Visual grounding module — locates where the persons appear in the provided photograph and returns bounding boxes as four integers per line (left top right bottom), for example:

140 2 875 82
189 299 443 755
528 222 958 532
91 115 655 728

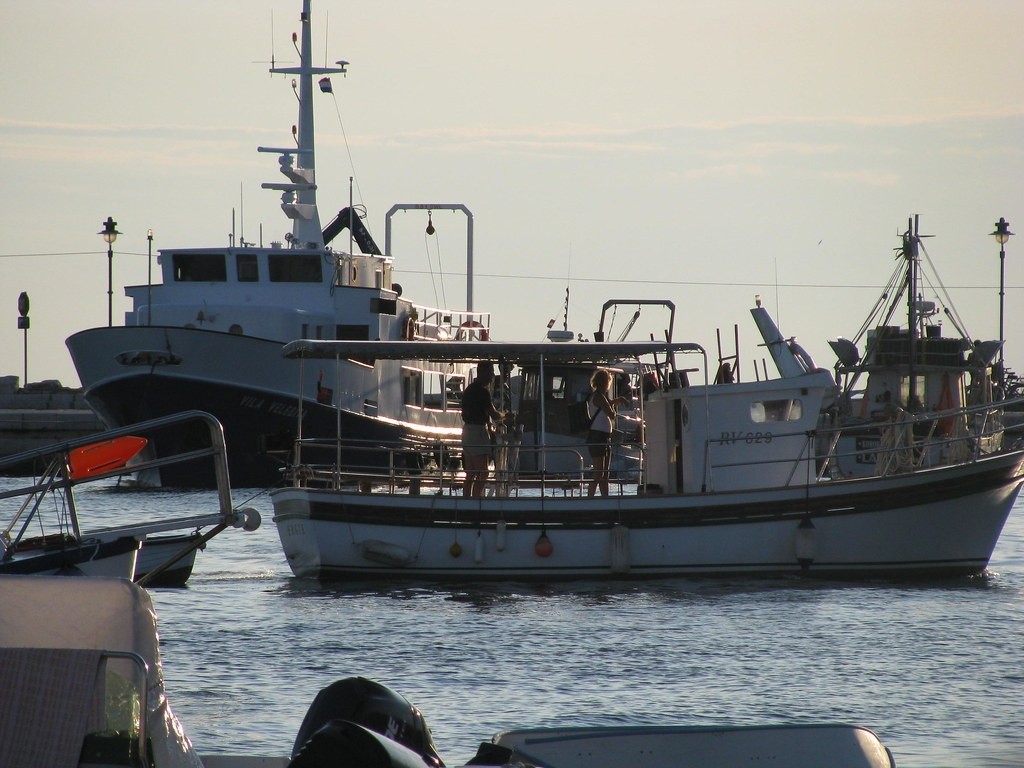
880 391 905 422
717 363 735 383
587 369 630 497
460 361 507 498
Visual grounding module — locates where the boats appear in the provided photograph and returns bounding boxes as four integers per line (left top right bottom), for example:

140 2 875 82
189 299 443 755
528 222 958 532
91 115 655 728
0 411 895 768
65 0 521 487
269 215 1024 581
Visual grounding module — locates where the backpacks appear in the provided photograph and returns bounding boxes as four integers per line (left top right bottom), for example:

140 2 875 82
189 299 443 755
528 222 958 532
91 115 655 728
567 393 601 433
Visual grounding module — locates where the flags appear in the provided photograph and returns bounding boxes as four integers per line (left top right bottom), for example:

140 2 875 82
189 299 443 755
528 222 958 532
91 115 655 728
319 76 334 93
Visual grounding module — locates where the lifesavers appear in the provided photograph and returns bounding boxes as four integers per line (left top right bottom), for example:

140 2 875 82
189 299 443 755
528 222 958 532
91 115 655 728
456 321 489 340
403 317 416 340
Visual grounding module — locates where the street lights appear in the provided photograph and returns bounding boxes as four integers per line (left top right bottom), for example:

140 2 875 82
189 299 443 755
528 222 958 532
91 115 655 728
987 218 1015 364
94 217 124 325
